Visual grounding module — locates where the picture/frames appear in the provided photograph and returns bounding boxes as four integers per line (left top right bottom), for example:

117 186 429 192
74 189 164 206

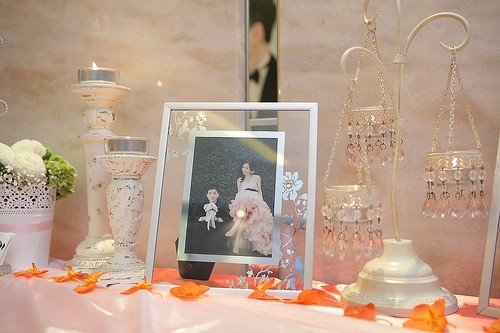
177 131 285 264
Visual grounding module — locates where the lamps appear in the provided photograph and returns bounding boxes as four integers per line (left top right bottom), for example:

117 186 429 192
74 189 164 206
321 0 487 319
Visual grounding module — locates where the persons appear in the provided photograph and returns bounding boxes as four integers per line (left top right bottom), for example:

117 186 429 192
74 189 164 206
190 186 232 255
224 160 263 255
248 0 278 130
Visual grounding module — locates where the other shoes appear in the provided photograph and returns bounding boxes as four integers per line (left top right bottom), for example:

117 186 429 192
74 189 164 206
233 242 239 255
225 229 237 237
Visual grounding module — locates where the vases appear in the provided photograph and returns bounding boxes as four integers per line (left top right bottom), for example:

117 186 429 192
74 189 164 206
0 184 57 272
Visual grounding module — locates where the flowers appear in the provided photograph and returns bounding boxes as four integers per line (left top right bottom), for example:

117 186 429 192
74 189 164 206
0 139 77 200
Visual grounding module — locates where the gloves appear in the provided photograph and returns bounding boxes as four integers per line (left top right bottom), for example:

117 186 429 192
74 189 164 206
203 203 218 212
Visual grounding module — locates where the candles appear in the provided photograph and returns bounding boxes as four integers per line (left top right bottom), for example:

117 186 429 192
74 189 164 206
77 61 118 84
107 137 148 154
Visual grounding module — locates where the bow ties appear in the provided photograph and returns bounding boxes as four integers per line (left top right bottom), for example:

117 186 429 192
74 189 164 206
250 70 259 82
